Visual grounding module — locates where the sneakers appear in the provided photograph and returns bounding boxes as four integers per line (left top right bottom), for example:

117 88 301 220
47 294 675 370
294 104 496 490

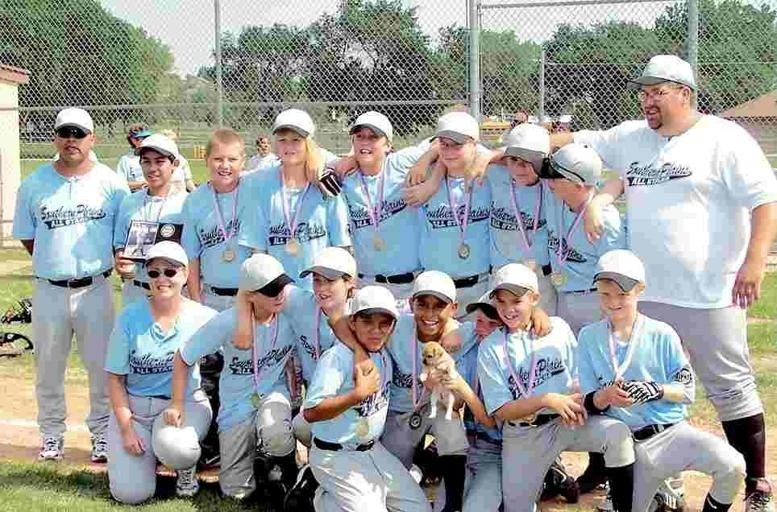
89 433 110 464
546 453 614 509
197 443 221 469
655 478 689 510
742 476 771 510
421 446 443 487
36 436 67 463
175 464 201 501
251 455 318 510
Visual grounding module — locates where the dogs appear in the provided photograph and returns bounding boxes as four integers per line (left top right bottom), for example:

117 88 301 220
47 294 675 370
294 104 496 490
419 341 456 421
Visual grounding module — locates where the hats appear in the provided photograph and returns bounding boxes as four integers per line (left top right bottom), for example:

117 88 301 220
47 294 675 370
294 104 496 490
299 246 356 281
591 248 647 293
348 284 401 322
143 240 189 270
239 253 295 298
626 54 699 96
466 263 539 319
410 270 458 308
128 128 178 163
54 108 94 136
428 112 481 144
272 108 315 140
500 122 601 187
348 111 394 143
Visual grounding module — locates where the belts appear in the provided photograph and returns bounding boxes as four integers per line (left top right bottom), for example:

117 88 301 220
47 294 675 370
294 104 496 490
134 280 150 290
313 436 384 452
488 264 552 278
357 273 414 284
453 273 479 288
48 268 114 288
631 423 671 444
509 412 560 428
211 288 237 296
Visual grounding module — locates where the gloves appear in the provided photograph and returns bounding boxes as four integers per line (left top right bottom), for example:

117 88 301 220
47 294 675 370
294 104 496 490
317 166 345 199
622 378 664 408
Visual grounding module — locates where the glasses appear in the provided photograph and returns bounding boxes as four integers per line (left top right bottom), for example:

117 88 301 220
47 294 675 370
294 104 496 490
145 266 183 277
58 127 87 139
636 86 684 102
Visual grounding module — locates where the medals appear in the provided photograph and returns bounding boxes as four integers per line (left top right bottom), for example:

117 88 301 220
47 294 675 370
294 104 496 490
605 312 642 409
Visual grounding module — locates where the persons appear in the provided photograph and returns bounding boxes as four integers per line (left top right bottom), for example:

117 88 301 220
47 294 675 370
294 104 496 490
553 52 776 512
9 108 635 512
575 250 748 512
